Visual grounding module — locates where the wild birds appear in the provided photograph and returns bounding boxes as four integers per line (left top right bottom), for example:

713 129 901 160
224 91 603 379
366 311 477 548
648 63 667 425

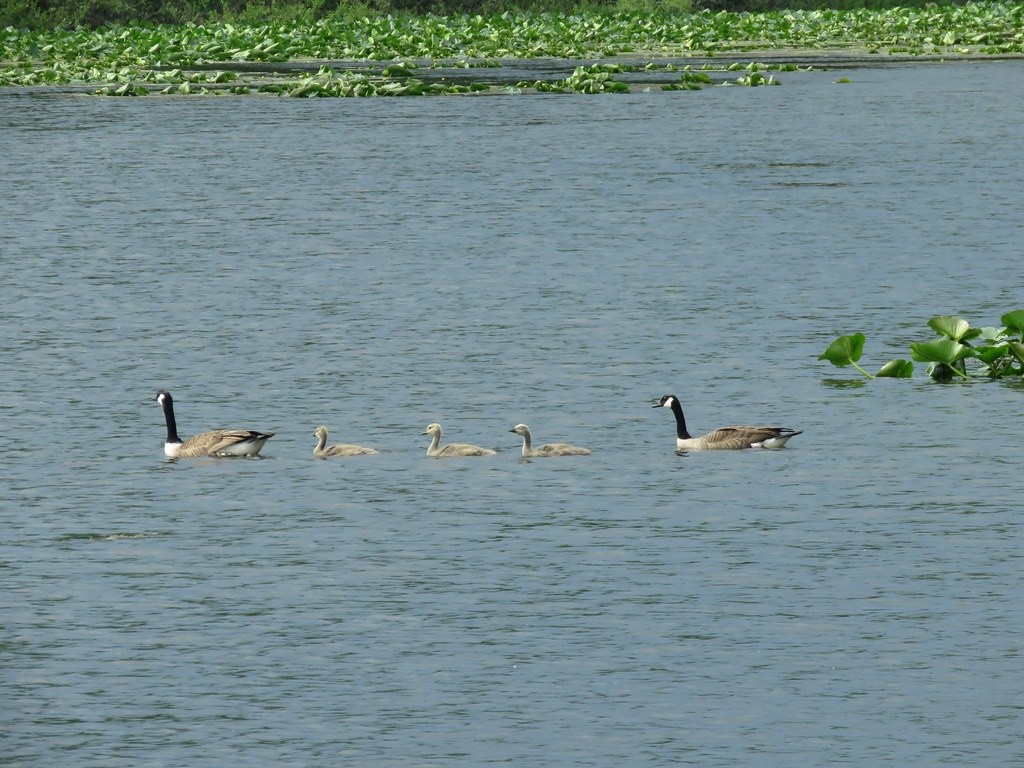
652 393 803 450
152 390 275 456
312 426 379 456
508 423 591 457
421 423 496 456
